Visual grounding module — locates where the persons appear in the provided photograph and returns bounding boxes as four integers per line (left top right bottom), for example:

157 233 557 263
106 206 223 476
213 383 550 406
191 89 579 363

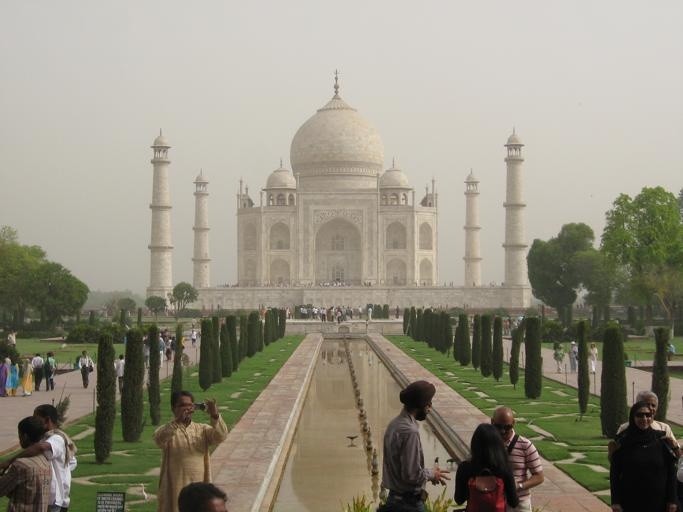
153 387 228 512
454 423 519 512
667 343 675 360
0 317 201 398
488 407 545 512
554 338 600 375
1 415 53 512
468 311 521 336
177 480 228 512
32 404 78 512
606 390 683 511
286 304 437 323
375 379 451 512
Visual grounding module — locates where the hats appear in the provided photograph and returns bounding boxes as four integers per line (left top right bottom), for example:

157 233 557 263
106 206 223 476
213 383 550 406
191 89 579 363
400 380 436 408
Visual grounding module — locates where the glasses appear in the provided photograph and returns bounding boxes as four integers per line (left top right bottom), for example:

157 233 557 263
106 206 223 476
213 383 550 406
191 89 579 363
493 423 514 430
634 411 653 418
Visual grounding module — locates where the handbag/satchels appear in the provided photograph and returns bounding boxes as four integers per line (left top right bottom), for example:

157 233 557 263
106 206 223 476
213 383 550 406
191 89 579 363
88 367 93 373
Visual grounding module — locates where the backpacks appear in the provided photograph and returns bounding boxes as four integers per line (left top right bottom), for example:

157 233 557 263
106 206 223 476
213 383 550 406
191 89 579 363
465 468 508 512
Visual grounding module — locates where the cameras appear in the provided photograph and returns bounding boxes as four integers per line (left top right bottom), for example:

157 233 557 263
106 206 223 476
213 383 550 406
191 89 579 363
191 403 205 410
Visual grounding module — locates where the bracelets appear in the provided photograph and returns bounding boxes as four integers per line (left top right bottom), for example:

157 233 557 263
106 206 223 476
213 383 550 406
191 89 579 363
519 482 524 490
